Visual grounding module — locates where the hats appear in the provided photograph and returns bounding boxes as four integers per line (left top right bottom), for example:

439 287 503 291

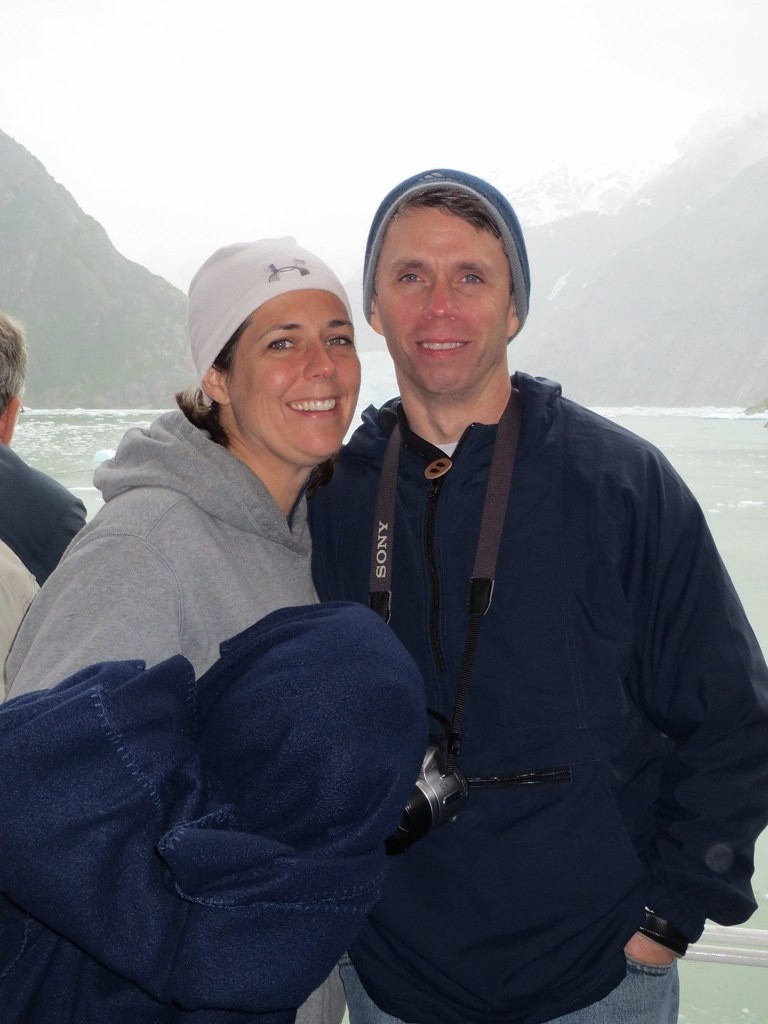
362 167 530 346
188 234 354 404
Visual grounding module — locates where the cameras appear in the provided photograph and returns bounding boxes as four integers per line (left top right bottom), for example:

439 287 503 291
392 746 469 848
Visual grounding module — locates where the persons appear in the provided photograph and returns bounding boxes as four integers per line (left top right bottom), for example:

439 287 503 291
304 169 768 1024
0 320 88 695
1 237 430 1024
